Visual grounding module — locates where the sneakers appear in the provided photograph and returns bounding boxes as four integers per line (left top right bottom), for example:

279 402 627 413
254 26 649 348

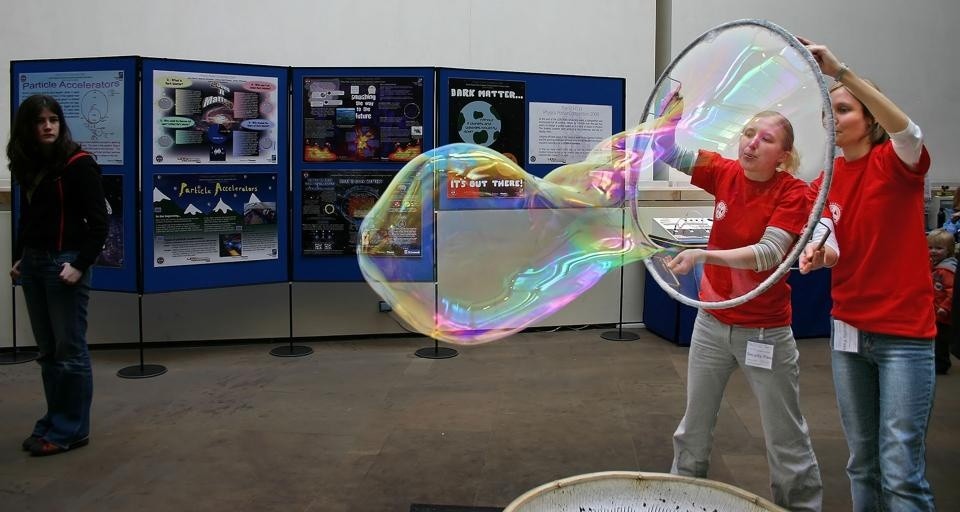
21 432 88 456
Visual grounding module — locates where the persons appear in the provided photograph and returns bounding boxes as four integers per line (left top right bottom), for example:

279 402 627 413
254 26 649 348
791 38 937 512
950 186 960 360
925 229 958 375
5 94 115 458
653 83 824 512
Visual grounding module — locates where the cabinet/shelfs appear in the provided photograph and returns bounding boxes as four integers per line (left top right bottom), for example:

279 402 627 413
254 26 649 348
643 249 832 349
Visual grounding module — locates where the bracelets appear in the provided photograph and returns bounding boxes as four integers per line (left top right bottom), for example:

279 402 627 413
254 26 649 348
834 62 847 84
